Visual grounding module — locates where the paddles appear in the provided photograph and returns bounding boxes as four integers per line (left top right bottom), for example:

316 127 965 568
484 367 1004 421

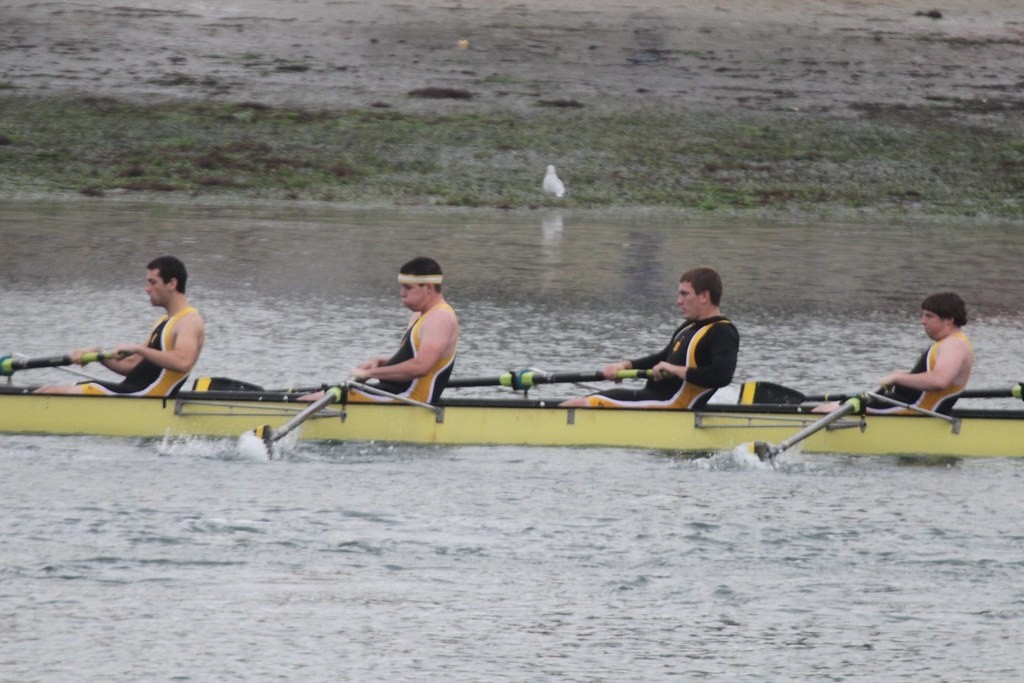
1 347 126 377
736 379 1023 404
253 375 367 462
747 384 893 464
444 365 671 390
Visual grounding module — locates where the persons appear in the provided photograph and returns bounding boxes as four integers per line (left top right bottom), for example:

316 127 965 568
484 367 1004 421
559 265 740 410
33 255 205 397
809 292 974 418
296 257 459 407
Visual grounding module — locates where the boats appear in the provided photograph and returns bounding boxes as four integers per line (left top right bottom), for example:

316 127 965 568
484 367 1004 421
0 383 1024 462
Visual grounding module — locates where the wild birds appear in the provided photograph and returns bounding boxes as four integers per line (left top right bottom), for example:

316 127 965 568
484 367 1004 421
542 165 565 200
